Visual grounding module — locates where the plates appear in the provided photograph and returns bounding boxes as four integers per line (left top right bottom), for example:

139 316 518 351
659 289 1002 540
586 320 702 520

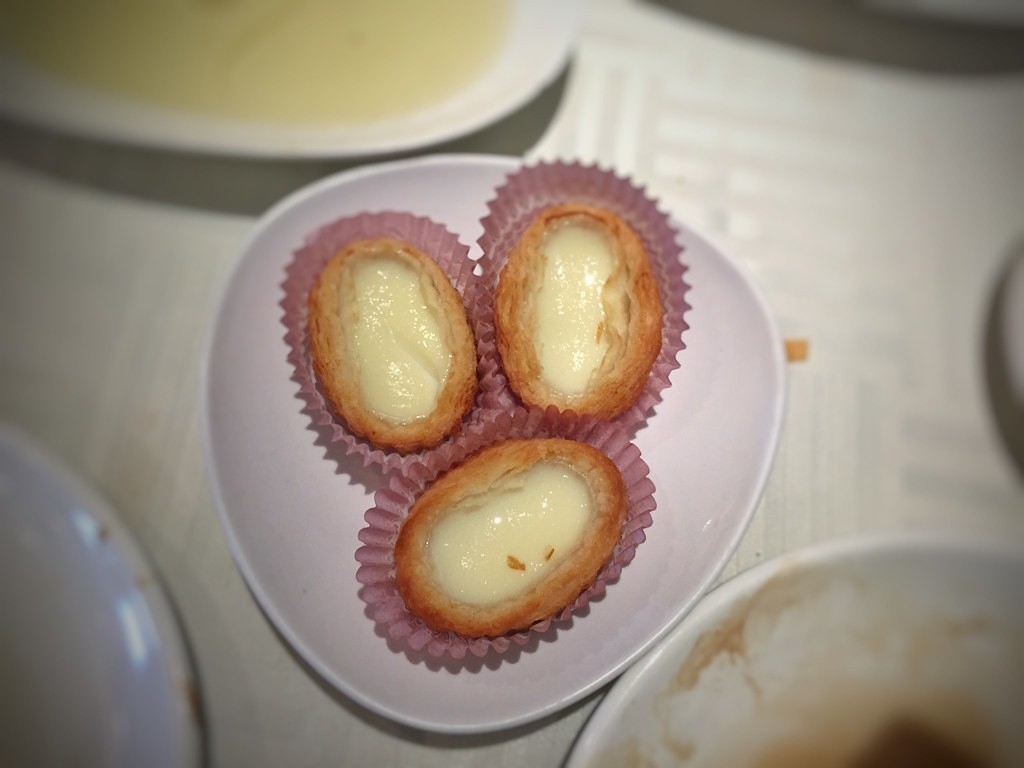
0 426 207 768
0 0 575 160
201 154 788 735
563 532 1024 768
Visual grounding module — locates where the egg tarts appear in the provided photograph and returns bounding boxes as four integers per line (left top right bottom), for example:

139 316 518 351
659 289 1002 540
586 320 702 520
305 236 475 451
394 439 627 638
493 209 660 421
